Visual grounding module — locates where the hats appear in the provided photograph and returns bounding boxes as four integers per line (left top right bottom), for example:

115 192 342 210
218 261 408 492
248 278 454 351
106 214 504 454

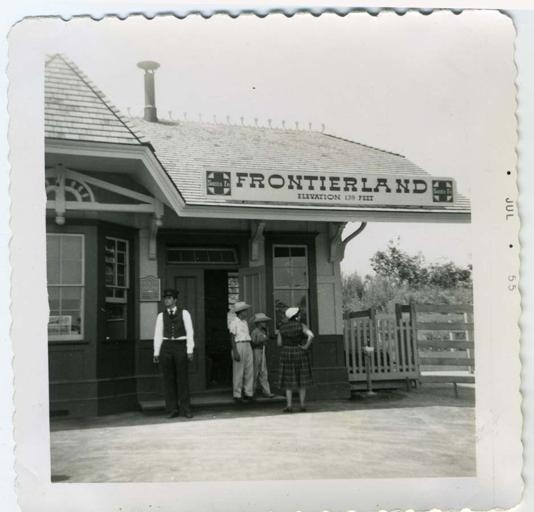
232 301 251 314
253 312 272 323
163 289 179 298
285 307 300 319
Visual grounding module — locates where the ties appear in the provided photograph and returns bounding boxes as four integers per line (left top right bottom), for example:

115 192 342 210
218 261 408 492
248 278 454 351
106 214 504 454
170 310 173 318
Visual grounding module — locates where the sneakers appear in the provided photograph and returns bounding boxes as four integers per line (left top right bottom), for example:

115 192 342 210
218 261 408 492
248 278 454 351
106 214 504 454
283 407 307 412
262 393 275 398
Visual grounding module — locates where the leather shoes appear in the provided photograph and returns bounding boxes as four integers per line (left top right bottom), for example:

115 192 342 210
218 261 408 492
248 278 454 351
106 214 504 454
183 408 193 418
167 409 179 418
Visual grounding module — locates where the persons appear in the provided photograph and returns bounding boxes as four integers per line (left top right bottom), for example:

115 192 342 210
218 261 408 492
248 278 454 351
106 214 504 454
228 300 274 404
272 305 317 413
152 288 197 420
